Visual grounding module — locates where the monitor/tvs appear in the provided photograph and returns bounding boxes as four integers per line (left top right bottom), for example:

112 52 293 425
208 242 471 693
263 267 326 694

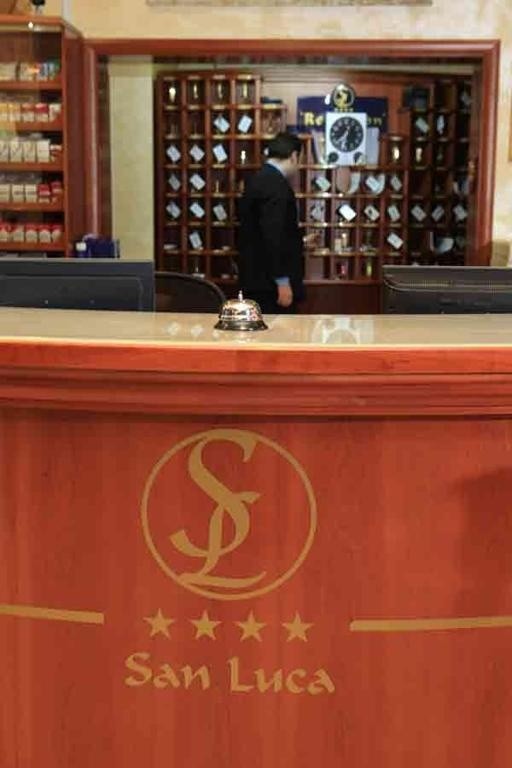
381 262 512 315
0 259 158 311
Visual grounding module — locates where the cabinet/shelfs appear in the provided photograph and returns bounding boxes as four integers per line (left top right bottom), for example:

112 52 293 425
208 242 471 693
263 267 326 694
0 13 87 259
156 71 479 281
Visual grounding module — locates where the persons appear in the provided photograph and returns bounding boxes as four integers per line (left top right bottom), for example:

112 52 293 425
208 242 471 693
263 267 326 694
239 132 305 314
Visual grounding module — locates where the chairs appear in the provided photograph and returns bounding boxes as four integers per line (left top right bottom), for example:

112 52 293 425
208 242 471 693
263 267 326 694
154 272 227 313
